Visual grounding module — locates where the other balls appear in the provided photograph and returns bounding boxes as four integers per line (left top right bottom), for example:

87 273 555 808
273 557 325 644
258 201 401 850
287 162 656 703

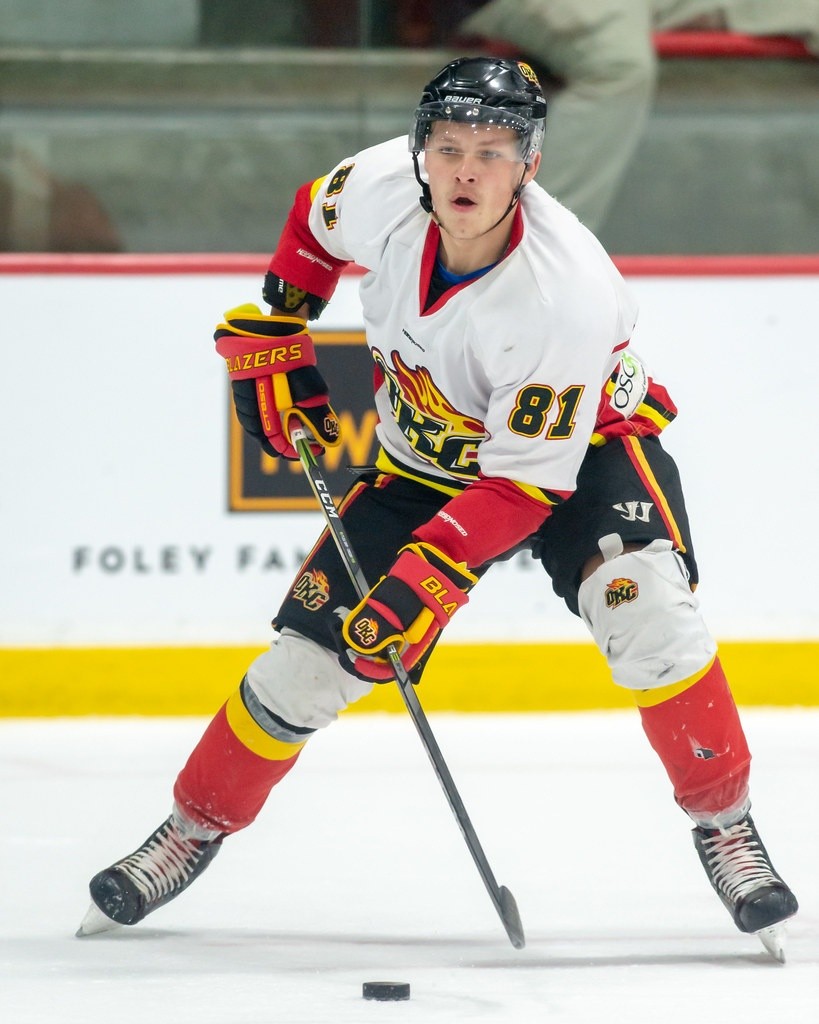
361 980 413 1002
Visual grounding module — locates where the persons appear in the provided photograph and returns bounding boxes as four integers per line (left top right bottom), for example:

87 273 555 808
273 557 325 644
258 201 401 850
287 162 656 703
423 1 659 235
78 53 802 967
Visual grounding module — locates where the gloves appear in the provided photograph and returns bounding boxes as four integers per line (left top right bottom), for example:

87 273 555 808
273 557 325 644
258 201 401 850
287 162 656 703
327 541 479 685
214 302 342 461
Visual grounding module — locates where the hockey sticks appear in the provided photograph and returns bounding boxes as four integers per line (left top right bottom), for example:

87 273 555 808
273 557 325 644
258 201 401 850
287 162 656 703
287 411 527 952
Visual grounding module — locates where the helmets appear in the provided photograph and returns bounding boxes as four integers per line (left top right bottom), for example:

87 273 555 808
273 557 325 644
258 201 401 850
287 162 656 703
411 55 548 168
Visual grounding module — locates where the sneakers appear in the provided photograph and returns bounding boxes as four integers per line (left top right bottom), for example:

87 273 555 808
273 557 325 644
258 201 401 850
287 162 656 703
75 814 222 937
691 813 799 964
